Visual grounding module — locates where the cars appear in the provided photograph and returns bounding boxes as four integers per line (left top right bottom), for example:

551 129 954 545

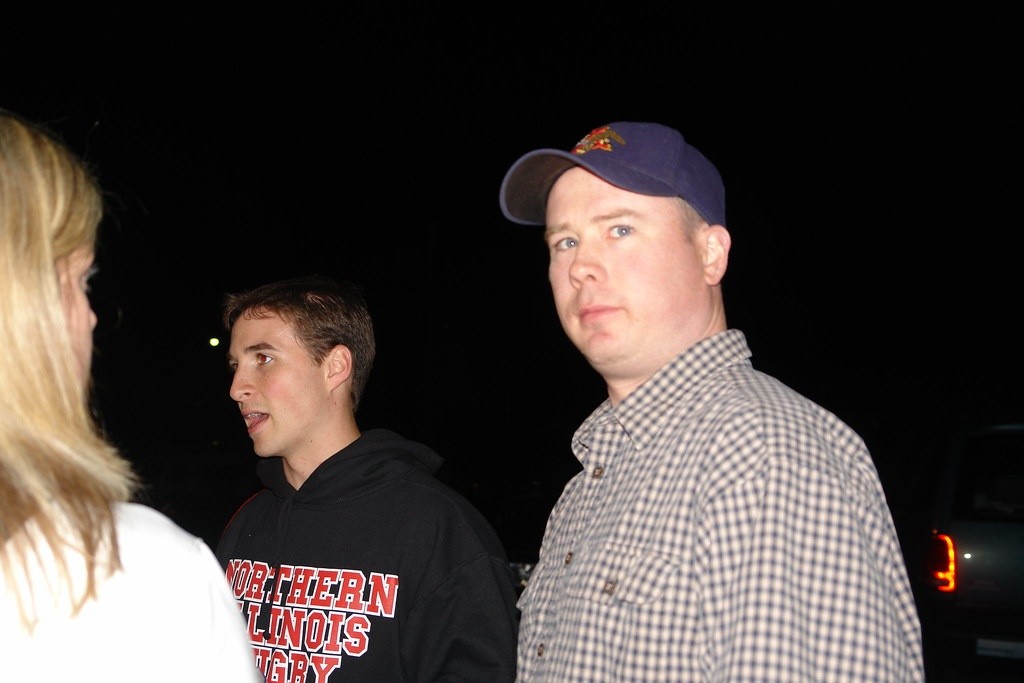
926 423 1024 666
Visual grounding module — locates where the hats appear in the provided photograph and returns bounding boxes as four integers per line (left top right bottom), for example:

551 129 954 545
501 124 726 225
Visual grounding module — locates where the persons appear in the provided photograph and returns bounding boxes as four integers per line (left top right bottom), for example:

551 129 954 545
499 122 925 683
0 115 269 683
216 274 520 683
